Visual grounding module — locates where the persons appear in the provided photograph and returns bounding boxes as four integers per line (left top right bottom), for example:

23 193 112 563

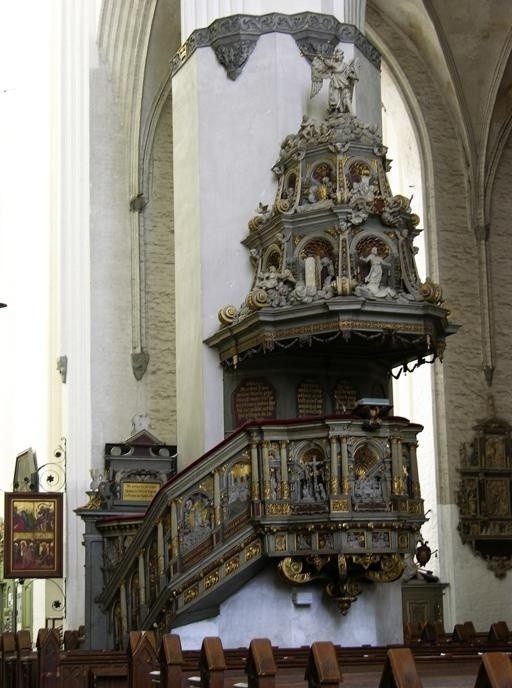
318 48 360 113
320 256 334 286
359 246 392 291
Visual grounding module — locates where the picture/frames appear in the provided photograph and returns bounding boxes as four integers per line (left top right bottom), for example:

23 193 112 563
4 492 62 578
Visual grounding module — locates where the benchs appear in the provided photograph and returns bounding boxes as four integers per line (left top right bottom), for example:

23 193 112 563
0 628 510 688
